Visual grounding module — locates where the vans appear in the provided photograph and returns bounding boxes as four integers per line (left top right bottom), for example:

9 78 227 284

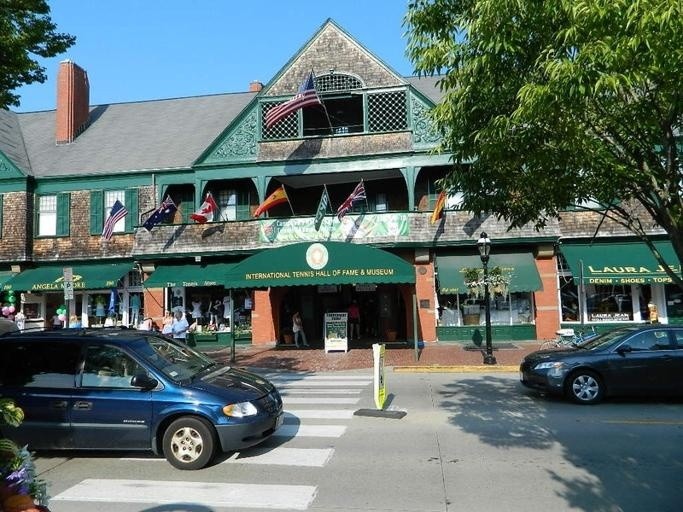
0 323 284 470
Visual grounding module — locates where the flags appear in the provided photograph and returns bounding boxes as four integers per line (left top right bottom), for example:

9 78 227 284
190 194 217 225
336 182 365 223
314 189 328 232
429 190 446 226
142 196 176 232
101 200 128 240
107 287 120 317
253 186 288 218
264 73 321 129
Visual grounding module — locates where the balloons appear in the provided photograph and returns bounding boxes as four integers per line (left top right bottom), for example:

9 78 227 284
1 289 15 316
56 303 68 320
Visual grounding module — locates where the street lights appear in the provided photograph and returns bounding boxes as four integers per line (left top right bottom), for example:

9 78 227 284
475 232 498 367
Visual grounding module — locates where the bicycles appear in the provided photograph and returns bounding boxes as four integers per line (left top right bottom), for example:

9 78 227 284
536 330 584 352
557 325 600 345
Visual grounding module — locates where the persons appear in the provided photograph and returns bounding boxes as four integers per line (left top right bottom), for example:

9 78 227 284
291 309 310 349
347 299 361 341
207 299 221 330
129 293 142 326
222 296 233 326
94 295 107 317
49 315 63 330
170 310 190 345
68 312 81 329
14 308 27 331
190 299 203 319
364 298 377 335
172 289 183 308
161 311 173 337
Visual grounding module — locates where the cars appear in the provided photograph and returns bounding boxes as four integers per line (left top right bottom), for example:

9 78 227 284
515 322 682 406
